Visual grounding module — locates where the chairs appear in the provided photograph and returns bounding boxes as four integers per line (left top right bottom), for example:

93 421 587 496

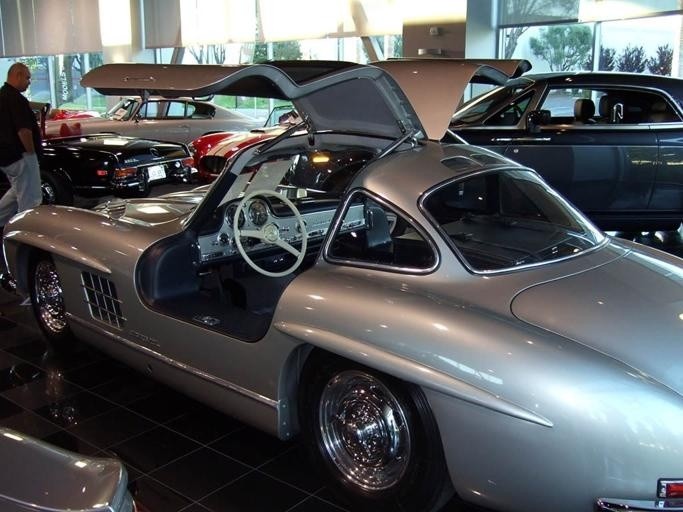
574 96 667 124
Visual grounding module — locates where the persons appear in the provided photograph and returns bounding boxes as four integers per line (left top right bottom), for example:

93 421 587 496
0 61 46 233
